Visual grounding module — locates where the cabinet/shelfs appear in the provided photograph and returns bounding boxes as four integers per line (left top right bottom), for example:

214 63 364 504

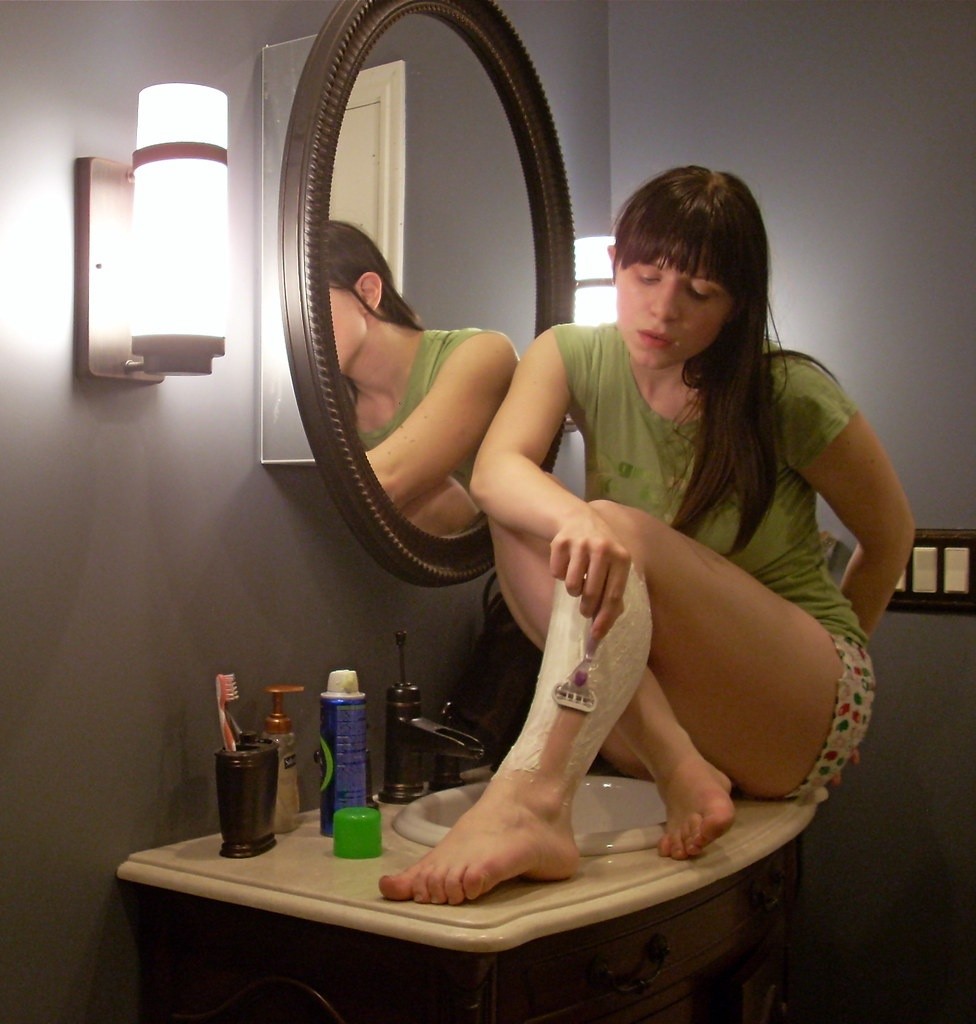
142 835 805 1024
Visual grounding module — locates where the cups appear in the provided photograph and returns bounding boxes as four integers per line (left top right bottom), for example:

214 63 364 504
214 739 280 858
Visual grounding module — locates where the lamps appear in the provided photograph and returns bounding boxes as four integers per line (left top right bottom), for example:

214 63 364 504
572 237 618 326
73 82 229 382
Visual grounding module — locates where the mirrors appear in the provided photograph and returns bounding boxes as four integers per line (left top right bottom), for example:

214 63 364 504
279 0 576 590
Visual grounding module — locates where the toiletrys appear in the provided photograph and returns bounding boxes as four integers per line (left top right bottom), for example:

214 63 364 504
262 680 306 834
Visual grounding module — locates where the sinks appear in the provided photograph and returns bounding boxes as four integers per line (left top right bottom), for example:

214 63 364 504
391 769 701 852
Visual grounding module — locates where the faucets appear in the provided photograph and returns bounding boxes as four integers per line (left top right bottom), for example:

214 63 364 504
378 628 484 806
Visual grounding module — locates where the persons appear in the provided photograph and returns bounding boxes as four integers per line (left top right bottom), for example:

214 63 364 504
377 163 917 904
328 221 517 540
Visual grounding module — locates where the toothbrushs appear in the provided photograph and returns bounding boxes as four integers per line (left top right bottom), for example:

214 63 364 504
214 671 240 753
